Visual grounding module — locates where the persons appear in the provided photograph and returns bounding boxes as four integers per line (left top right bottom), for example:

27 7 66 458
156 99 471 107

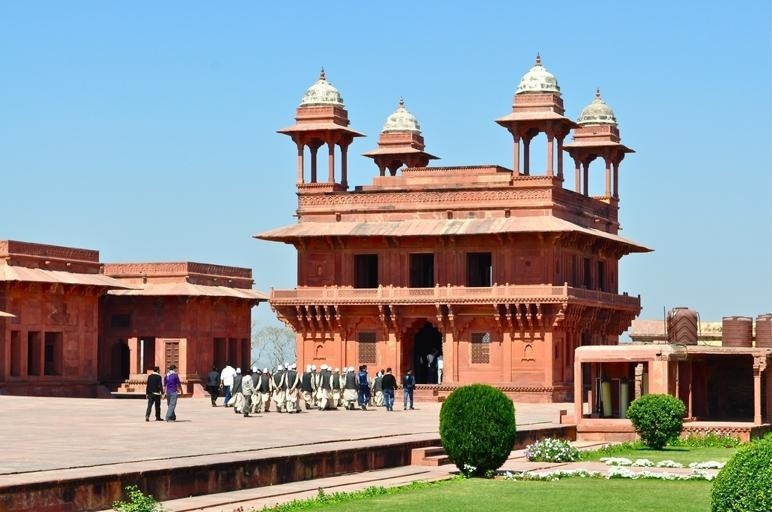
420 351 444 385
202 359 419 417
144 365 164 424
162 363 184 423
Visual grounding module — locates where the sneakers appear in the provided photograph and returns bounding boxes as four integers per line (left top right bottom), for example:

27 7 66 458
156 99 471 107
234 402 374 418
403 406 415 410
386 405 393 411
145 416 150 422
155 418 163 421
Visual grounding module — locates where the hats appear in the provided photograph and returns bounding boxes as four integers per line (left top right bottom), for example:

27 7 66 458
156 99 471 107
235 363 355 373
169 364 178 370
